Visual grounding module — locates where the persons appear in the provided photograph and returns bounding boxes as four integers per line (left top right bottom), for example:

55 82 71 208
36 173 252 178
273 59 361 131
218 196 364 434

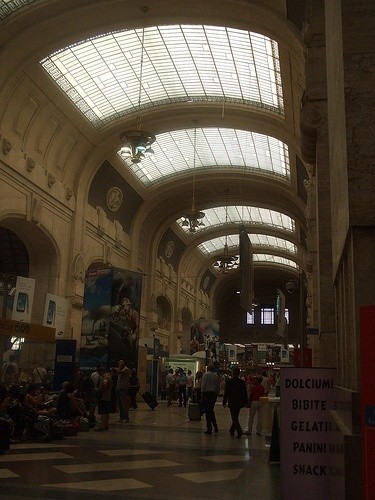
0 354 20 392
129 368 141 409
89 364 113 428
31 361 47 391
165 367 203 408
18 369 32 396
44 366 55 390
112 360 131 423
200 363 221 435
218 366 233 397
238 369 255 401
223 368 248 439
261 373 277 397
111 291 139 355
198 321 210 344
245 374 266 436
208 335 221 362
74 363 88 391
0 380 89 451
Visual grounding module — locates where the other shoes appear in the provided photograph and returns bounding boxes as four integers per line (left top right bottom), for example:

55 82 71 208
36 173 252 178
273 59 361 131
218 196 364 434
118 417 129 423
256 432 260 435
231 432 234 436
204 431 212 434
236 432 243 439
244 432 251 435
93 422 109 431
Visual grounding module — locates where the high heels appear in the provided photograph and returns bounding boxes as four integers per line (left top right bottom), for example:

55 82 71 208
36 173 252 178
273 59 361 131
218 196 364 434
12 433 27 441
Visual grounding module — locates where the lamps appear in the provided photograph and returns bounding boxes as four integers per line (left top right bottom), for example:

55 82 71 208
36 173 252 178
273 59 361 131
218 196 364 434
118 6 156 164
181 121 205 233
213 206 238 274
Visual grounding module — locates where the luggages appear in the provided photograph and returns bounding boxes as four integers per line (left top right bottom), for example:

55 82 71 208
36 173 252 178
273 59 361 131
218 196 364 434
138 392 158 411
188 391 202 421
